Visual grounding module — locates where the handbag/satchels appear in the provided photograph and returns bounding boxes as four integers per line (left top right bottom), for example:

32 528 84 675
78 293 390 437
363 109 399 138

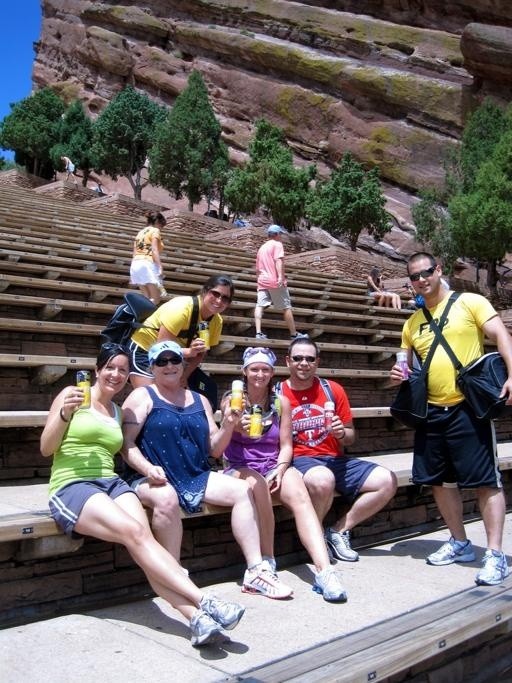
456 351 512 420
187 295 219 413
389 366 429 429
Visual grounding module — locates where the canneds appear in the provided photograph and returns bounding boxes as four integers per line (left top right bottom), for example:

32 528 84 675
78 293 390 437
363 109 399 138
396 351 410 381
324 401 336 431
199 320 210 351
76 370 92 409
231 380 244 412
248 404 262 440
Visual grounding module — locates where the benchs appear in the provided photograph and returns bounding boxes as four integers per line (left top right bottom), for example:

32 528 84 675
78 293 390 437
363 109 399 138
0 181 512 683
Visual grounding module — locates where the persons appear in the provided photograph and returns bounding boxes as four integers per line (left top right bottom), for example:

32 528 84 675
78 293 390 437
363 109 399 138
253 223 309 339
129 210 165 303
95 182 102 193
368 265 390 308
378 273 403 309
272 337 399 561
38 341 246 647
407 279 450 310
120 338 294 599
60 155 78 188
127 273 234 389
390 252 512 584
219 346 348 601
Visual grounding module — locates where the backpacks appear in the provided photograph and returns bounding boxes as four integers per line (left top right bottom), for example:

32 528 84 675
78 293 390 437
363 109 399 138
97 290 214 346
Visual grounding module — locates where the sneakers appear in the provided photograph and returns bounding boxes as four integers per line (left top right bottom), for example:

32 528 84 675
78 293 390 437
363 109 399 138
255 333 268 339
198 593 245 629
475 549 509 585
426 537 476 565
290 332 310 340
189 610 230 646
238 527 359 602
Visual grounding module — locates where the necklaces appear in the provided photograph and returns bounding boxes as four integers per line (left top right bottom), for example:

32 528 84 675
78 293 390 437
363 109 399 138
246 392 269 402
160 386 182 404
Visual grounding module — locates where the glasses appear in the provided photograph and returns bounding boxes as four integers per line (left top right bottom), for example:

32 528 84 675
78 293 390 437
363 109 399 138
245 347 274 359
291 356 316 362
210 290 232 305
151 355 182 367
409 266 437 282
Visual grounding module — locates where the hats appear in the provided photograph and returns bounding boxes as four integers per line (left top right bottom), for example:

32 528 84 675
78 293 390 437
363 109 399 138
241 347 277 368
267 225 286 235
149 341 184 366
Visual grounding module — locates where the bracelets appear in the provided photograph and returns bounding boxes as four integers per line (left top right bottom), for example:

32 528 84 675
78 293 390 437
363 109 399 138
336 431 345 439
280 466 285 475
60 408 71 423
